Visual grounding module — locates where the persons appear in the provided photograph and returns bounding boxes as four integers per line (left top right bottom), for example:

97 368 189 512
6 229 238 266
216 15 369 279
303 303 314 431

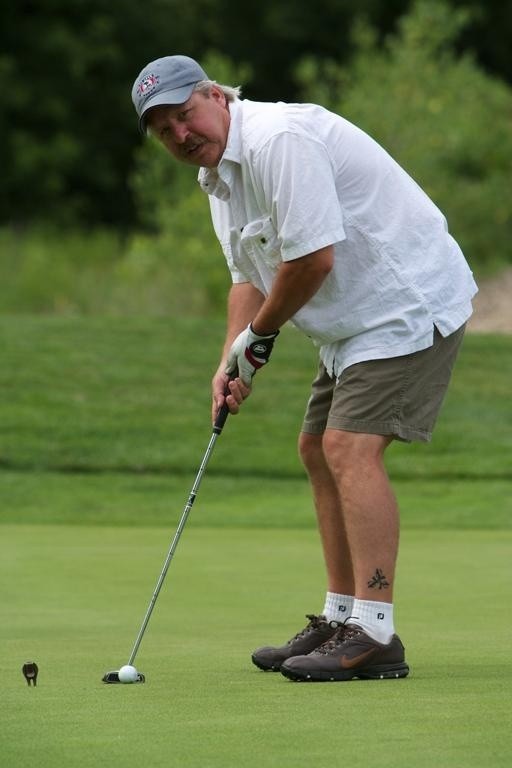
132 55 479 682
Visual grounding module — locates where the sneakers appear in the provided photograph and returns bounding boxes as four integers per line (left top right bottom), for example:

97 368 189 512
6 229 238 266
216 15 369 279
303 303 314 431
252 615 409 682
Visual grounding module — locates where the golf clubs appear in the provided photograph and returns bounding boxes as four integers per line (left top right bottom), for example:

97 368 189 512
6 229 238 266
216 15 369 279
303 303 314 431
102 367 239 684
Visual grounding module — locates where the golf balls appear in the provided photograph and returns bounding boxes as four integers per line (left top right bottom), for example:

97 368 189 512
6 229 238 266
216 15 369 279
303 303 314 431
118 665 137 683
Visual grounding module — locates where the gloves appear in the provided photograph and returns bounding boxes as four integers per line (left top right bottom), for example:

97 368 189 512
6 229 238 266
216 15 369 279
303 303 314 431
224 320 280 387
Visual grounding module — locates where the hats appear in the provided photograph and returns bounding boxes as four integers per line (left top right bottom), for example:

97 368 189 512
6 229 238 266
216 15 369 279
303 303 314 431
131 55 210 137
